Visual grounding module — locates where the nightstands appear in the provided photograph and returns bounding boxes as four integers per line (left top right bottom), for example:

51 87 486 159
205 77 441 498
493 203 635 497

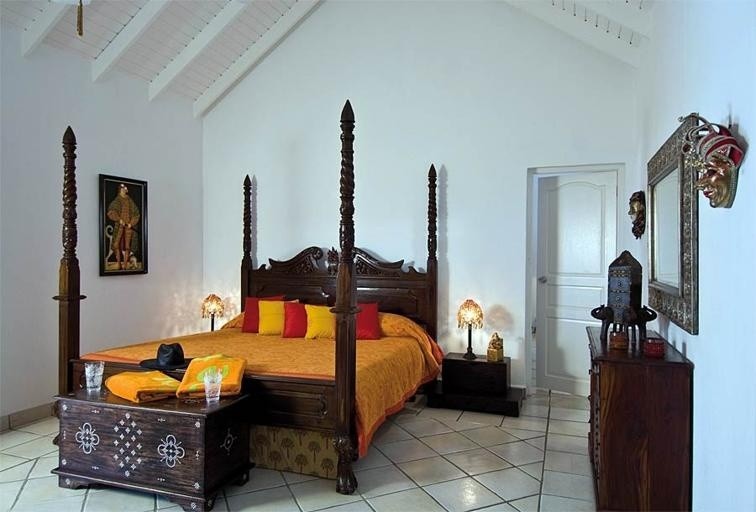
442 350 511 398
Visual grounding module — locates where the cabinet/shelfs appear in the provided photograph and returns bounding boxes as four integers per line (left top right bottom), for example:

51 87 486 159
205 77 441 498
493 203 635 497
607 249 642 324
583 324 694 512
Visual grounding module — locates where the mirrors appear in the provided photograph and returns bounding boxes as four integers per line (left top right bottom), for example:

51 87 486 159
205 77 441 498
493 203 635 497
645 112 701 334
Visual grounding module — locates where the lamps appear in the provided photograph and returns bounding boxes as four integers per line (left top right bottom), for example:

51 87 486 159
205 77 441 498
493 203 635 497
455 298 485 360
200 293 226 333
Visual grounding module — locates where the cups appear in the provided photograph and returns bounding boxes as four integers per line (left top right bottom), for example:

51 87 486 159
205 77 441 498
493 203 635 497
643 337 666 357
204 375 223 402
85 359 104 388
609 330 628 350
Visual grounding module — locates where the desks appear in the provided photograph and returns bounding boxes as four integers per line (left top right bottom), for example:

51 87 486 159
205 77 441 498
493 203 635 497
52 386 254 510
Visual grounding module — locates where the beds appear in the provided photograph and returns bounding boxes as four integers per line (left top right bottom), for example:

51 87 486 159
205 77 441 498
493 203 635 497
52 97 443 495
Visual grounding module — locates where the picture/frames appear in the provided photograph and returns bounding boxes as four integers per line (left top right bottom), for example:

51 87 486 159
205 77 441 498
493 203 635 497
98 172 148 278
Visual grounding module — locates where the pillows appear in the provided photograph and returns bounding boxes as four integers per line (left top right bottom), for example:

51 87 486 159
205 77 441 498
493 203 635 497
240 293 381 340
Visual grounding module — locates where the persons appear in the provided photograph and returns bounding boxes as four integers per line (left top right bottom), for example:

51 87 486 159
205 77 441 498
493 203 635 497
107 184 139 269
625 200 645 222
692 158 733 209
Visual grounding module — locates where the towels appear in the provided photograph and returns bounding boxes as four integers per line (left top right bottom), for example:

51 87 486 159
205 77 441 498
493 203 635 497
175 353 249 402
103 366 184 407
